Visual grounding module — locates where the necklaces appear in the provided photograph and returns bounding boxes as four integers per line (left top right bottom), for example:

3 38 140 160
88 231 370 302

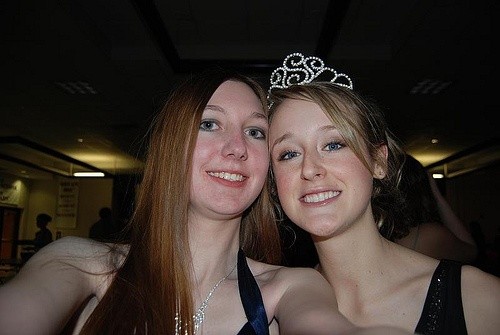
174 260 237 334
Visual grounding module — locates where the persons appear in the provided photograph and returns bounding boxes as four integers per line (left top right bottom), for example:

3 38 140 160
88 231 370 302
17 213 53 251
268 83 500 335
86 207 117 244
0 68 410 335
388 152 483 267
